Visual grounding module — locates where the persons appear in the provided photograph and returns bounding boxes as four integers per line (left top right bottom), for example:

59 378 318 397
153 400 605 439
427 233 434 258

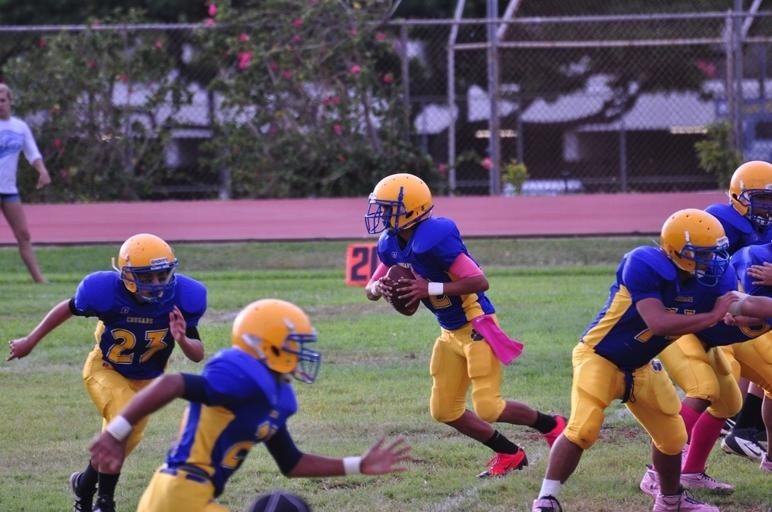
726 238 772 471
1 84 52 284
703 161 771 461
726 291 772 318
364 173 569 479
639 243 771 497
719 367 768 462
747 261 772 286
88 298 413 512
531 208 738 512
5 233 206 512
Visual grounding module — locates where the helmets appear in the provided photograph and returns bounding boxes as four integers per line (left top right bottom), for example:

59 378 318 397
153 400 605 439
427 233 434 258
364 172 432 234
661 208 731 286
118 233 178 308
232 299 321 385
728 160 772 232
252 493 309 512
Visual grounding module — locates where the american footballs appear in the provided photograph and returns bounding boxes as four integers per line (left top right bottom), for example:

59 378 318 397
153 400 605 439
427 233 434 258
386 265 419 315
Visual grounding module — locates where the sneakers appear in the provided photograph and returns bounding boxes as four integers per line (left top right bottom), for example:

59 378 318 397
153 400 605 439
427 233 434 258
721 431 768 460
654 494 718 512
760 453 772 474
680 468 736 496
639 464 661 498
720 418 736 436
69 470 95 512
94 496 116 511
540 416 566 449
532 495 562 512
476 448 527 478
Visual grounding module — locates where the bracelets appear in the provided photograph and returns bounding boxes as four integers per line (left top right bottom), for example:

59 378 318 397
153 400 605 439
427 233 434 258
425 282 445 297
104 414 133 444
369 280 379 298
342 456 362 476
